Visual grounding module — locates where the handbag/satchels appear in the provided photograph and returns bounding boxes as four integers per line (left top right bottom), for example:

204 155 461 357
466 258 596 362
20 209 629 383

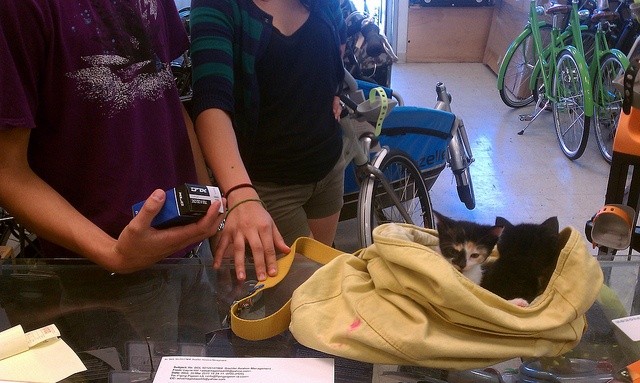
230 222 604 372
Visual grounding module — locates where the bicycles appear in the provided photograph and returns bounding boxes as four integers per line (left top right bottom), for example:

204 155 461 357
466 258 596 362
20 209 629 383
496 0 593 160
529 0 631 163
339 1 436 249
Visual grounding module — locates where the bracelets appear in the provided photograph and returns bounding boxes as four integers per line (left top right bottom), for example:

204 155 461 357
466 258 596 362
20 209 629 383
226 183 258 196
226 198 268 218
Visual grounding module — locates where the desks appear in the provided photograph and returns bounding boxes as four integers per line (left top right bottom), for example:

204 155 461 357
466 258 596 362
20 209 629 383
0 256 639 382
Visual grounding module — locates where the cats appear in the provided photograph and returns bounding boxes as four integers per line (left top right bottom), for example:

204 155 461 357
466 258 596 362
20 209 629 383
430 210 531 308
480 215 559 303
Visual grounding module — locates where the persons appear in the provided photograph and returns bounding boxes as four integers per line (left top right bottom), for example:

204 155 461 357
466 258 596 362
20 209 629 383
1 1 219 346
191 1 346 282
